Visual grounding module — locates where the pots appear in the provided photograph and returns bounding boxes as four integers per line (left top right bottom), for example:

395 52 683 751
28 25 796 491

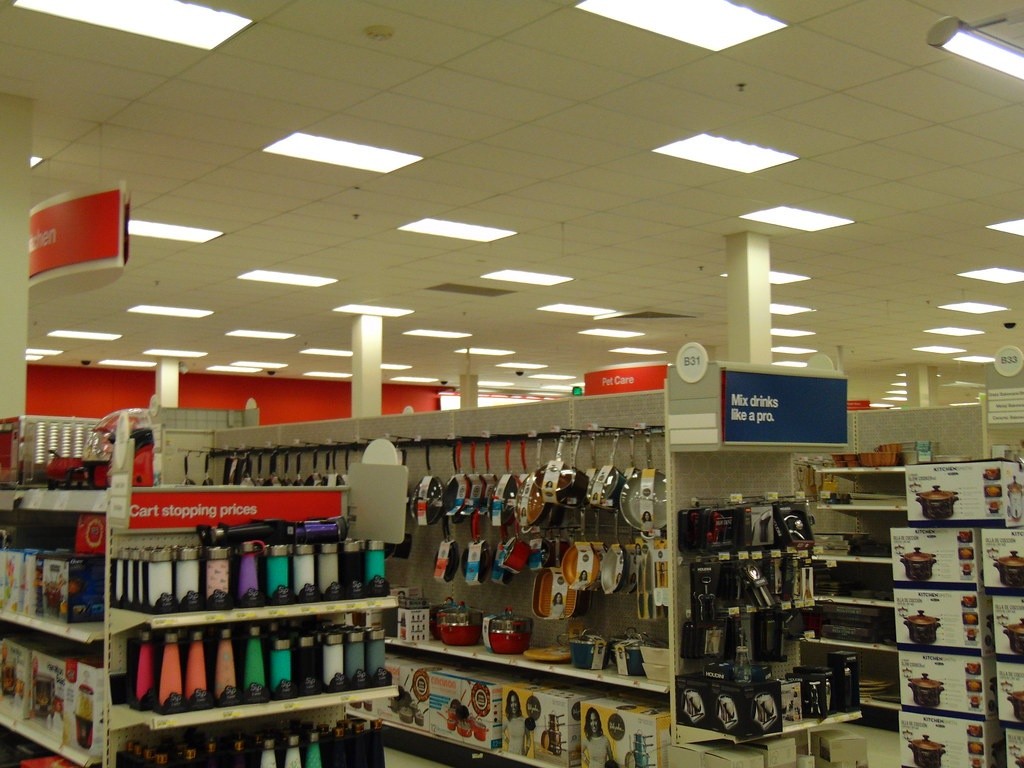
1002 618 1024 654
633 734 656 768
388 674 429 726
1006 476 1024 521
1007 691 1024 720
384 432 667 676
900 547 937 580
903 610 941 644
184 449 350 486
916 486 959 519
437 689 489 741
541 713 567 755
908 735 946 768
992 551 1024 586
907 673 944 707
350 701 372 711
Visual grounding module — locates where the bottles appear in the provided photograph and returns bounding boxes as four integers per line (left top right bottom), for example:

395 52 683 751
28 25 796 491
136 619 386 706
115 540 384 605
123 718 385 768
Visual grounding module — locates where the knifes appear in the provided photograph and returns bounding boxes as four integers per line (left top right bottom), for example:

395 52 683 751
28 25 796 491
638 561 644 617
646 551 653 619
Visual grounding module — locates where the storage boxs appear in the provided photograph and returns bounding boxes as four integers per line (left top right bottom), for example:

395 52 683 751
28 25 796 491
0 548 106 624
675 651 862 739
346 652 672 768
19 755 79 768
0 636 104 755
890 457 1024 768
346 586 430 642
668 731 869 768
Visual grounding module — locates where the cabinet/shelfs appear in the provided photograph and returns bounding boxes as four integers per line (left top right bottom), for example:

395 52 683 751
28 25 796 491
0 413 398 768
794 393 988 733
161 341 867 768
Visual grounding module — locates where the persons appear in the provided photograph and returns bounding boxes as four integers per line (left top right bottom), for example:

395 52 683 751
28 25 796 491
582 707 613 768
502 689 531 756
